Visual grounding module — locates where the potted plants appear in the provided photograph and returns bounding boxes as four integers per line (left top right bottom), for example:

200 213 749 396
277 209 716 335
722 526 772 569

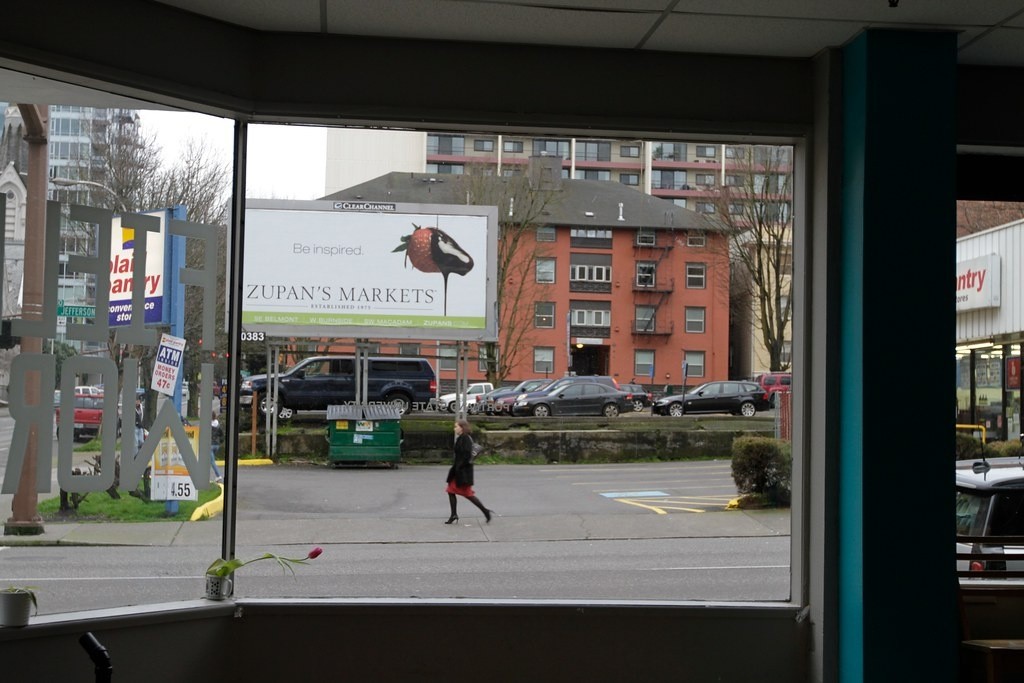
0 585 38 627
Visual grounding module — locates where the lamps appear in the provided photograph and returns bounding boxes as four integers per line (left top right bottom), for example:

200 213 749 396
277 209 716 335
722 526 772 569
956 338 995 351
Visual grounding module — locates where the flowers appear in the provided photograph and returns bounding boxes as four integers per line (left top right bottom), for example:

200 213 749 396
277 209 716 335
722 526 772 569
207 547 323 577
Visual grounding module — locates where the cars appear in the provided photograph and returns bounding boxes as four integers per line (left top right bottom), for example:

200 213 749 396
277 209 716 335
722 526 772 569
619 383 652 412
515 376 620 401
475 380 553 416
652 380 770 417
957 466 1024 581
512 382 633 417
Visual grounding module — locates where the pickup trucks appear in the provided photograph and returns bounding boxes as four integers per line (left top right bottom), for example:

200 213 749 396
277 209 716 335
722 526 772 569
55 394 104 441
439 383 494 413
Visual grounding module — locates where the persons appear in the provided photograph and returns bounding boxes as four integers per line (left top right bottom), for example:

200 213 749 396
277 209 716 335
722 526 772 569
132 392 145 456
444 420 495 524
208 409 225 481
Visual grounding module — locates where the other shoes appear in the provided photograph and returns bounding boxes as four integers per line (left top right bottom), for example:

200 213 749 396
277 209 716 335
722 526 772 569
214 476 222 482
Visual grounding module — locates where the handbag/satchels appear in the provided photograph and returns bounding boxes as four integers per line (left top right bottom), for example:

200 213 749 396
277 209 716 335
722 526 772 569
467 433 484 465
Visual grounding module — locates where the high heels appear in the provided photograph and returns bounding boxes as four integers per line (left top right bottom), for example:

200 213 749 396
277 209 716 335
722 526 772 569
486 509 495 523
445 515 459 524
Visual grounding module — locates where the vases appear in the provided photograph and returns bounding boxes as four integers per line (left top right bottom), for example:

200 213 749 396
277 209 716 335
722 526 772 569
205 574 231 599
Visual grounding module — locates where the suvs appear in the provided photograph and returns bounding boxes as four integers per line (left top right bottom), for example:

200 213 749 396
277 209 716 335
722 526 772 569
756 374 791 409
238 356 438 419
